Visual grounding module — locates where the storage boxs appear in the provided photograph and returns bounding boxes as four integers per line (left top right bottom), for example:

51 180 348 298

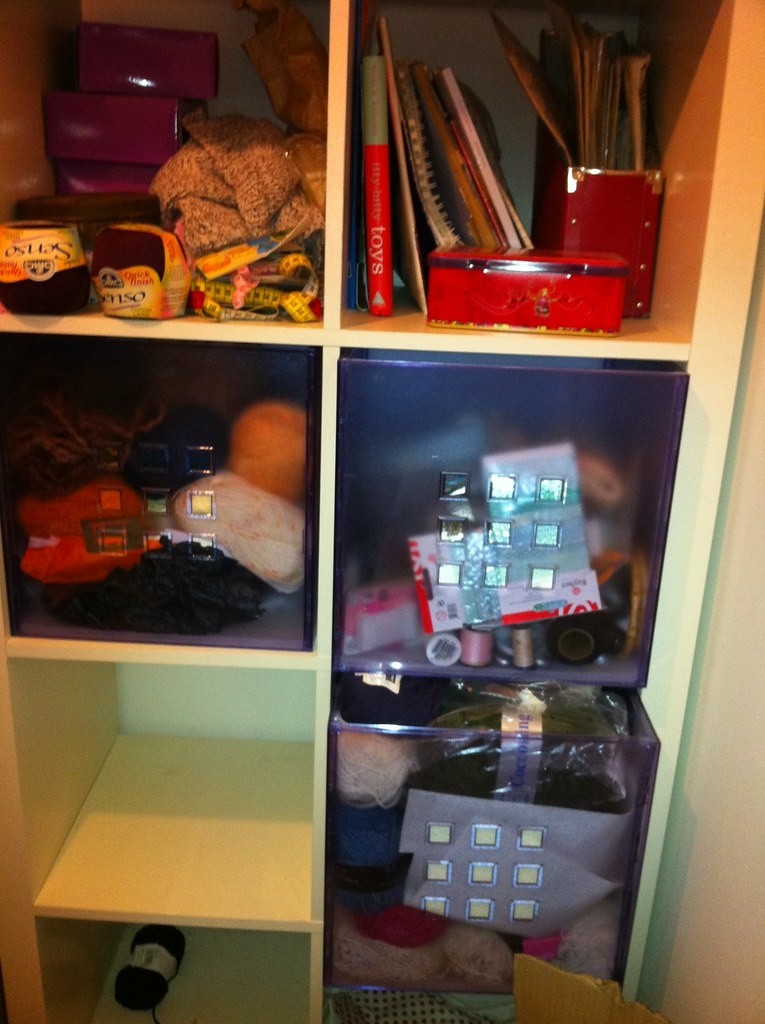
427 242 632 333
54 160 163 198
539 159 666 320
75 24 220 101
45 95 197 161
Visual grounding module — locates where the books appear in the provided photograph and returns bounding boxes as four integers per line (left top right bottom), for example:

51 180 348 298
345 1 651 318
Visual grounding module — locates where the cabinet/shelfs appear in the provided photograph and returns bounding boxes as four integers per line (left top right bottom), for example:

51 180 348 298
0 1 764 1024
